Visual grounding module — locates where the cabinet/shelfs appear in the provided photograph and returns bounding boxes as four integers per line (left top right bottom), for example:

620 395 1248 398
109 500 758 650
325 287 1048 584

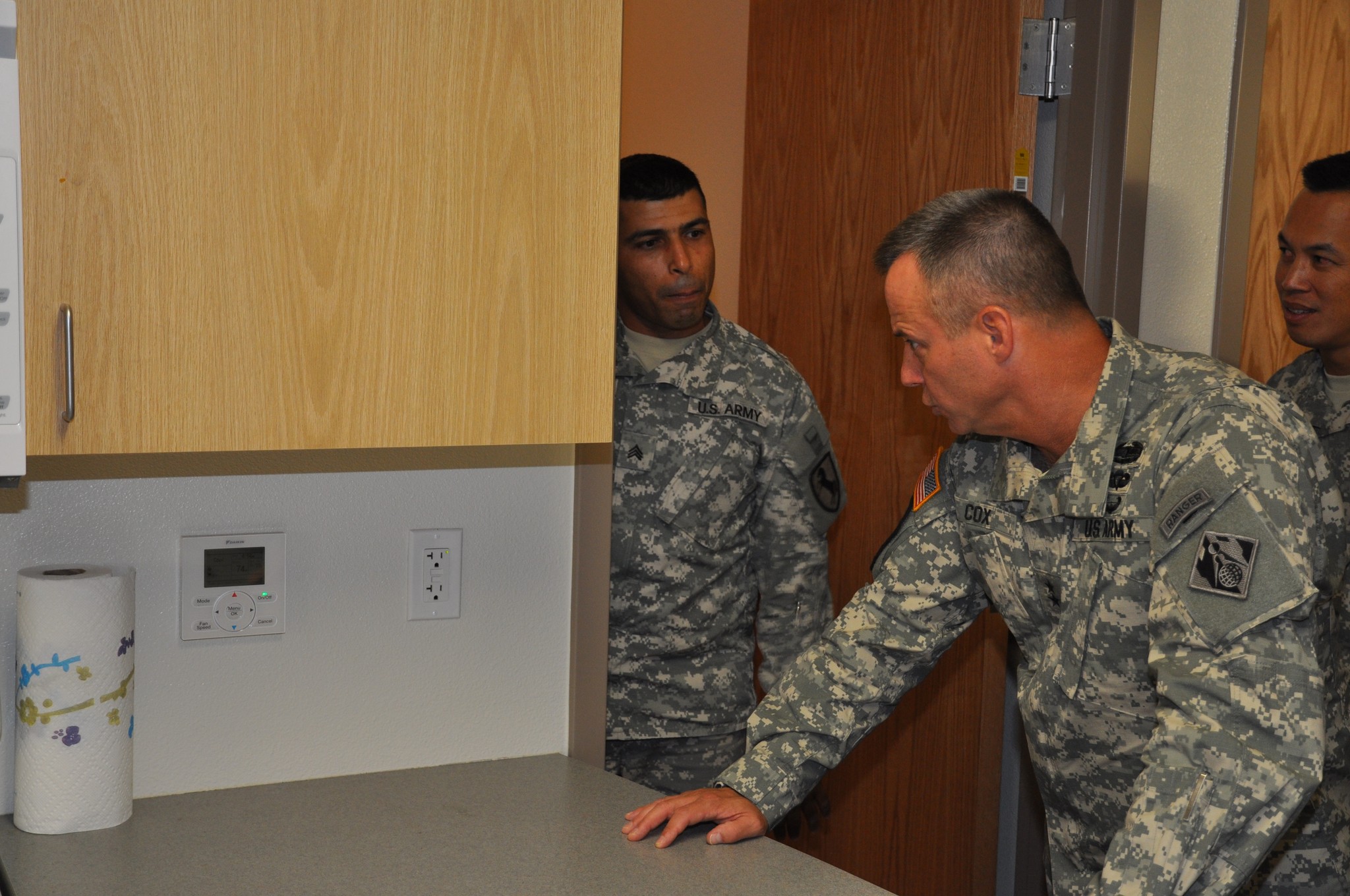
15 0 623 455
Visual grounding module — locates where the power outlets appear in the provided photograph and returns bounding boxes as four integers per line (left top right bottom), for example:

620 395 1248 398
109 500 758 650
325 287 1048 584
406 528 462 619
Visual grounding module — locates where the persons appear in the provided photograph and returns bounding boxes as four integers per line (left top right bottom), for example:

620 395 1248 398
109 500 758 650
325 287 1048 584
1260 154 1350 510
606 154 846 797
620 186 1350 896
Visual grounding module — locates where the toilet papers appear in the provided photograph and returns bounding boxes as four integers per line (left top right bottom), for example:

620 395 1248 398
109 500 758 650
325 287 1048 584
10 564 141 835
